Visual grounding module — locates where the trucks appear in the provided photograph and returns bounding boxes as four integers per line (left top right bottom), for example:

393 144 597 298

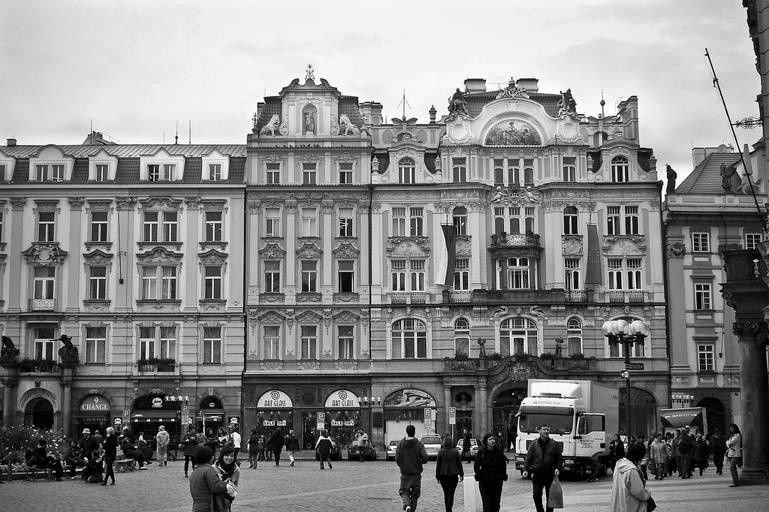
660 407 708 439
512 377 621 485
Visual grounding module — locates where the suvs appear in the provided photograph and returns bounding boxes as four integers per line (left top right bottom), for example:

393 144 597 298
418 434 444 460
455 437 480 459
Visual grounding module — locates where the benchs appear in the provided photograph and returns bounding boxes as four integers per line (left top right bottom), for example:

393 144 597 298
22 466 57 481
112 456 136 473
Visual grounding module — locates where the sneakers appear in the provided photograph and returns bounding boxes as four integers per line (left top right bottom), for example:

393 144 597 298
100 481 108 486
111 479 116 485
405 506 411 512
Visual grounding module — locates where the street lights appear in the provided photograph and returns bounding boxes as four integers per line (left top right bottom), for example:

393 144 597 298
356 395 382 443
670 394 695 407
600 316 652 453
163 394 189 458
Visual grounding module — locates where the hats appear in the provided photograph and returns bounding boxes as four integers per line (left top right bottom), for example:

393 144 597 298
159 424 165 430
82 428 90 434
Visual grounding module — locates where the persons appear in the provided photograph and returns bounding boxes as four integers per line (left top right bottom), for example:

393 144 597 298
523 424 563 512
315 430 333 469
474 433 508 512
497 432 505 453
610 442 651 512
395 425 429 512
436 427 472 512
354 428 369 462
610 419 744 489
32 412 300 511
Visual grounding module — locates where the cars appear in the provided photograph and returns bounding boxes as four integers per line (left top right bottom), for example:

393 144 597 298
313 438 344 461
386 439 399 459
346 438 378 461
619 434 629 452
